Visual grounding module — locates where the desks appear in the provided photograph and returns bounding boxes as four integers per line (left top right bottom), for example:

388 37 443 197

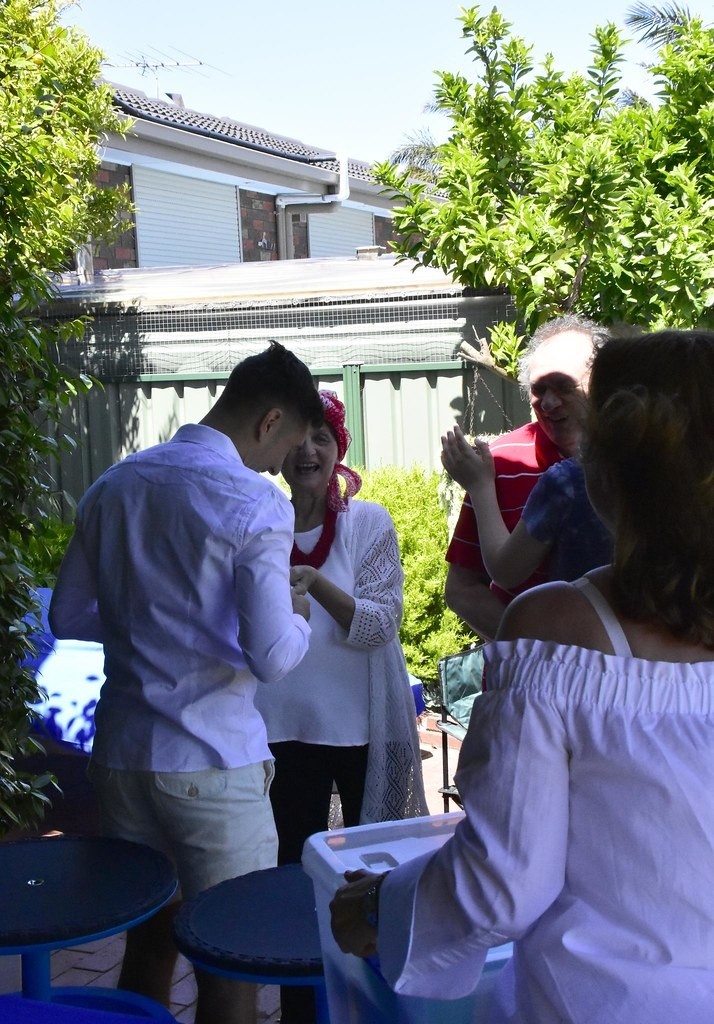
0 835 332 1024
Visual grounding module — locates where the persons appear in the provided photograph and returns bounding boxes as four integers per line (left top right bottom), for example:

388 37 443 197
47 341 328 1024
252 391 431 1024
327 328 714 1024
444 317 613 640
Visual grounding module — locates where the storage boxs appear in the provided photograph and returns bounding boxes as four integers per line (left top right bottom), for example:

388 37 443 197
300 810 514 1024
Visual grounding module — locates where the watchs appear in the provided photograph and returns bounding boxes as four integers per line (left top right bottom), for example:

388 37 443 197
365 869 389 931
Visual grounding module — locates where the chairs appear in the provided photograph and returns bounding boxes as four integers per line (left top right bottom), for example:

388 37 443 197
435 643 485 813
